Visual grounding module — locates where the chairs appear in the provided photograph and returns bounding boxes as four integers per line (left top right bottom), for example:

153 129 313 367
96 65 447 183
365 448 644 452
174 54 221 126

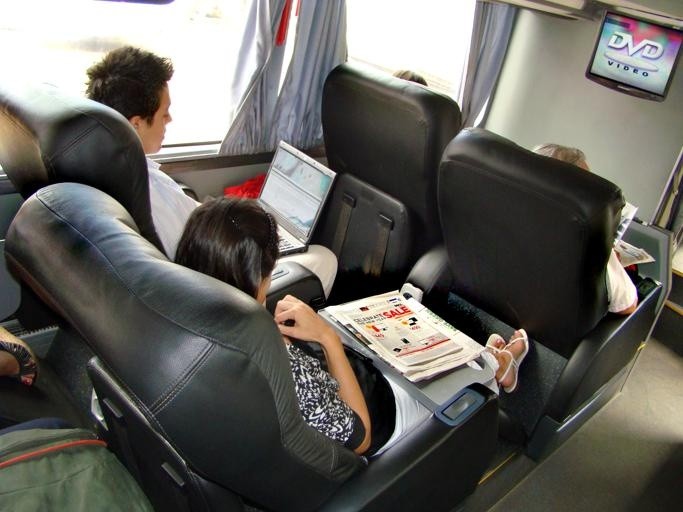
437 121 663 464
3 182 506 512
319 60 463 313
0 73 171 264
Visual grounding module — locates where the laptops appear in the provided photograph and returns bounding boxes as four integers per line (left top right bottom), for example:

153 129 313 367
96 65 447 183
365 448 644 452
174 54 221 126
256 140 337 260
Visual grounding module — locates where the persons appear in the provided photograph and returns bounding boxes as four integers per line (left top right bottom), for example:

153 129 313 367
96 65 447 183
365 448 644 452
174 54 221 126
532 143 638 314
81 44 338 300
0 340 36 388
171 196 528 462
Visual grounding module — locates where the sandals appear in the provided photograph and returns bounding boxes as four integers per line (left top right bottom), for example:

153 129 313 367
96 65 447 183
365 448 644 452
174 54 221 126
498 328 530 394
0 325 40 387
485 333 506 390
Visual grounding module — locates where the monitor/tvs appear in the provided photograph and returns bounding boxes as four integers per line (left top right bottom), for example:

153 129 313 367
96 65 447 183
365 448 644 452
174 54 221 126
584 8 683 102
